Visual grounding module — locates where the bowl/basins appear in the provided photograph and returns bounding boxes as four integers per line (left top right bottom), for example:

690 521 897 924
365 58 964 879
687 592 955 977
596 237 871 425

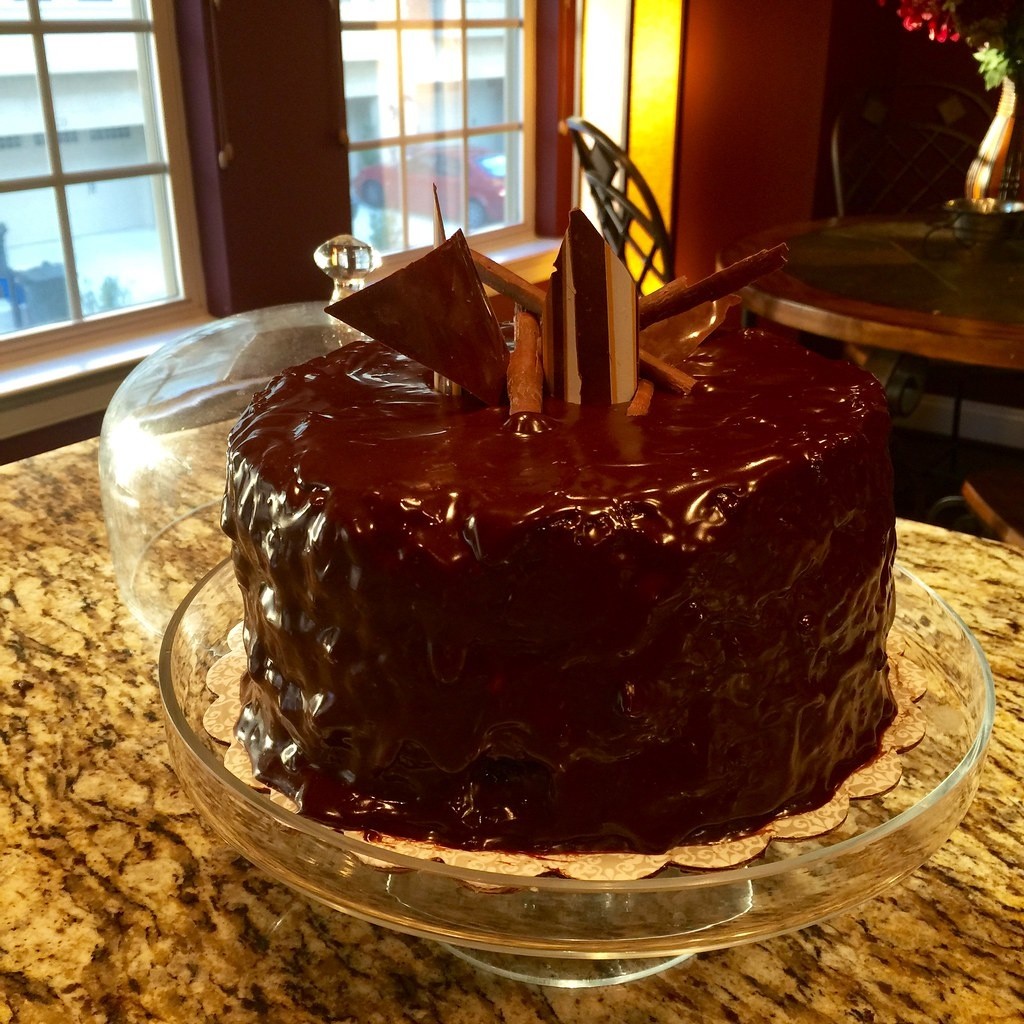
944 198 1024 242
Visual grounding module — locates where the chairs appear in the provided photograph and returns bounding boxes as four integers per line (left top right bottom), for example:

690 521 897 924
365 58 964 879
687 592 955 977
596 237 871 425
830 81 1015 218
566 115 676 297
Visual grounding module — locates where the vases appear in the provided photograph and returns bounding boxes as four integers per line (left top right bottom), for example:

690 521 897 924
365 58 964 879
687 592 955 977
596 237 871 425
963 78 1024 216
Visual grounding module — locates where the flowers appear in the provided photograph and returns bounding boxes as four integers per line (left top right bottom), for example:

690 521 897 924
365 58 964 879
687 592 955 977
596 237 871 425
877 0 1024 92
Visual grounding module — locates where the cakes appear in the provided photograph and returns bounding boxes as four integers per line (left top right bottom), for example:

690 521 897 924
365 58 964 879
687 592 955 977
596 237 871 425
220 116 896 858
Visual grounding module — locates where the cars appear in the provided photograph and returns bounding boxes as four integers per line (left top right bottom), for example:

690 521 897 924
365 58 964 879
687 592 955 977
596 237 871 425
354 144 507 228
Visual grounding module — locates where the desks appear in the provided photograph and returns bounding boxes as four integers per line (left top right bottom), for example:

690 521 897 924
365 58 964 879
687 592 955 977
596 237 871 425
0 420 1024 1024
715 210 1024 492
